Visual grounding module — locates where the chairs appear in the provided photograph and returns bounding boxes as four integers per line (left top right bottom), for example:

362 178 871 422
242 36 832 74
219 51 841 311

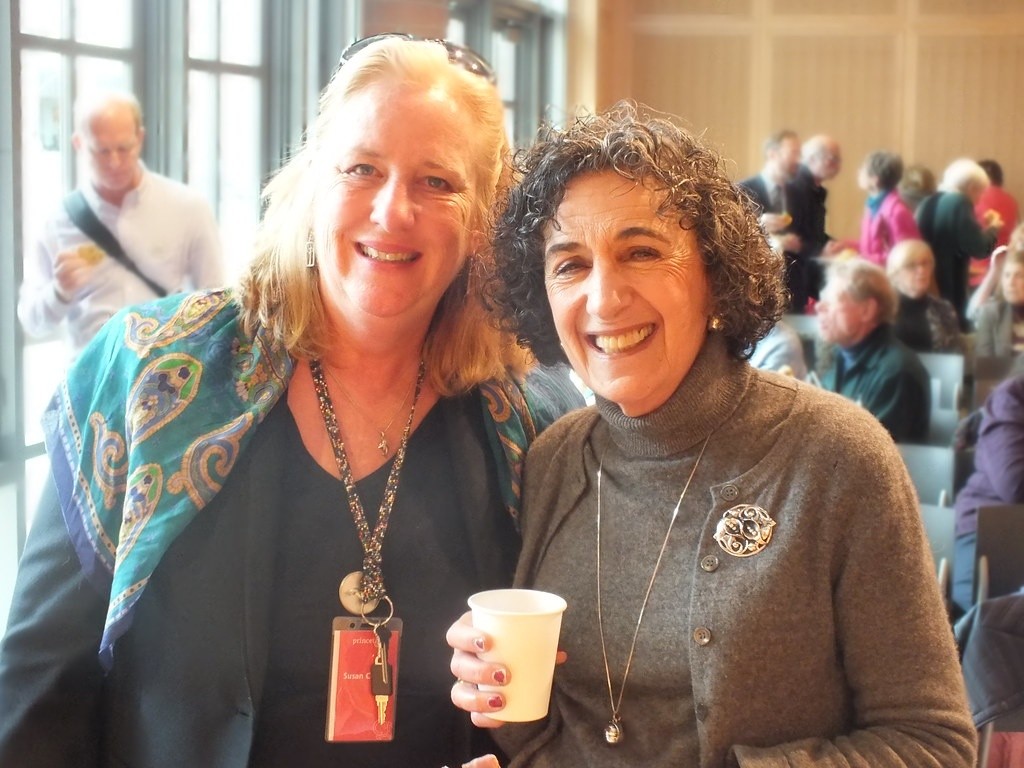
884 352 1024 768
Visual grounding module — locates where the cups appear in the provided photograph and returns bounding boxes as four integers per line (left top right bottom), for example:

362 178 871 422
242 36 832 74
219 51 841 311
467 589 567 721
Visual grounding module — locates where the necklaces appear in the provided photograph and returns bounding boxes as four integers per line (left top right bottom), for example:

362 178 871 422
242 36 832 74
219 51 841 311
597 421 715 745
323 360 421 458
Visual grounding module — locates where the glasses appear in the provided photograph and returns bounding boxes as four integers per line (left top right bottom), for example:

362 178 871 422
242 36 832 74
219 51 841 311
819 290 851 306
900 257 934 271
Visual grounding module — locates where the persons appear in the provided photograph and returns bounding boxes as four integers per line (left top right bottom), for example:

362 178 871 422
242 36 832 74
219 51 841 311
445 101 1024 768
0 32 555 768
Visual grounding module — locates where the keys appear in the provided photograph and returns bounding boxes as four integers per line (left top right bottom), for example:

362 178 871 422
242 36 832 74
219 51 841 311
362 595 395 723
